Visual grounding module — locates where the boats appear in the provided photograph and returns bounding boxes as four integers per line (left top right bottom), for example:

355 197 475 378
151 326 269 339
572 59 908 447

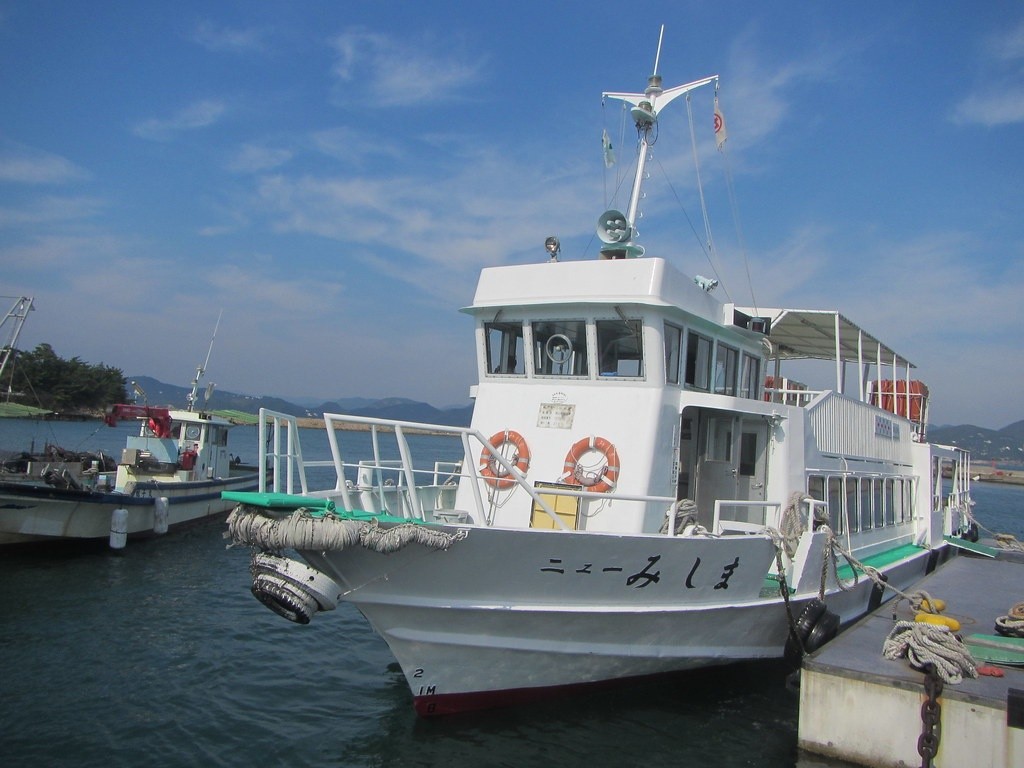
0 305 275 546
227 20 981 719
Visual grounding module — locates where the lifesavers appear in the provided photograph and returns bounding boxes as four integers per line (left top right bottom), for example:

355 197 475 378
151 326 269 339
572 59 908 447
783 596 840 672
563 437 620 496
969 523 978 541
479 430 530 490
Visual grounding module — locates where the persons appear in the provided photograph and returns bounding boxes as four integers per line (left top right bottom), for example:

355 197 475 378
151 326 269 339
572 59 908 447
494 355 517 373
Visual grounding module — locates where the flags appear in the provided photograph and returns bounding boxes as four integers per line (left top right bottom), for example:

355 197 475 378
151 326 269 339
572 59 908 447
713 97 728 153
602 129 616 169
